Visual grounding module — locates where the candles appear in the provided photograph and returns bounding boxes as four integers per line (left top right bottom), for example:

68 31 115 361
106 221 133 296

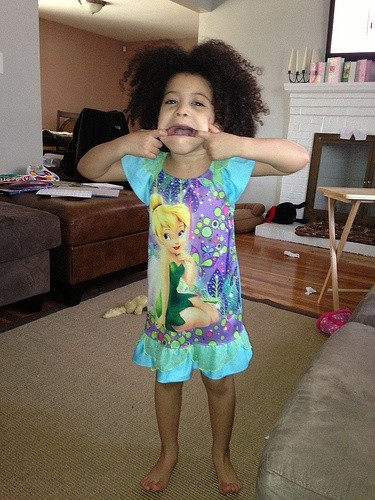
288 48 294 71
302 46 307 69
310 48 315 63
296 48 298 71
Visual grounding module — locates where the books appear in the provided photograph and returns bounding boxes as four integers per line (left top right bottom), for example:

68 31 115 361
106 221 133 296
50 186 120 198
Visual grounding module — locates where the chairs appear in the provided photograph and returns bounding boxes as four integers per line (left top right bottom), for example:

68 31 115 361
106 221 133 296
58 109 131 191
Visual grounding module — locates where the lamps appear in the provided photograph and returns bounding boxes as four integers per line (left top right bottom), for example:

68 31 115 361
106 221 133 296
77 0 107 14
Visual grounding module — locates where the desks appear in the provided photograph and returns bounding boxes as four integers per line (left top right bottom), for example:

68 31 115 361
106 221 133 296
317 186 375 311
42 130 74 182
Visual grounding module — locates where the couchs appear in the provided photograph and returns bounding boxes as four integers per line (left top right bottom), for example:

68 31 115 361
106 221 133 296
254 280 375 500
0 176 148 308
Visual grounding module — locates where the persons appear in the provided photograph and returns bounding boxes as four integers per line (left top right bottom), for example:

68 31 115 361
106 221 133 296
77 40 309 492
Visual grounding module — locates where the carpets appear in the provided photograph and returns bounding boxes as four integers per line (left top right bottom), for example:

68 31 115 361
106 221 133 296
0 278 328 500
